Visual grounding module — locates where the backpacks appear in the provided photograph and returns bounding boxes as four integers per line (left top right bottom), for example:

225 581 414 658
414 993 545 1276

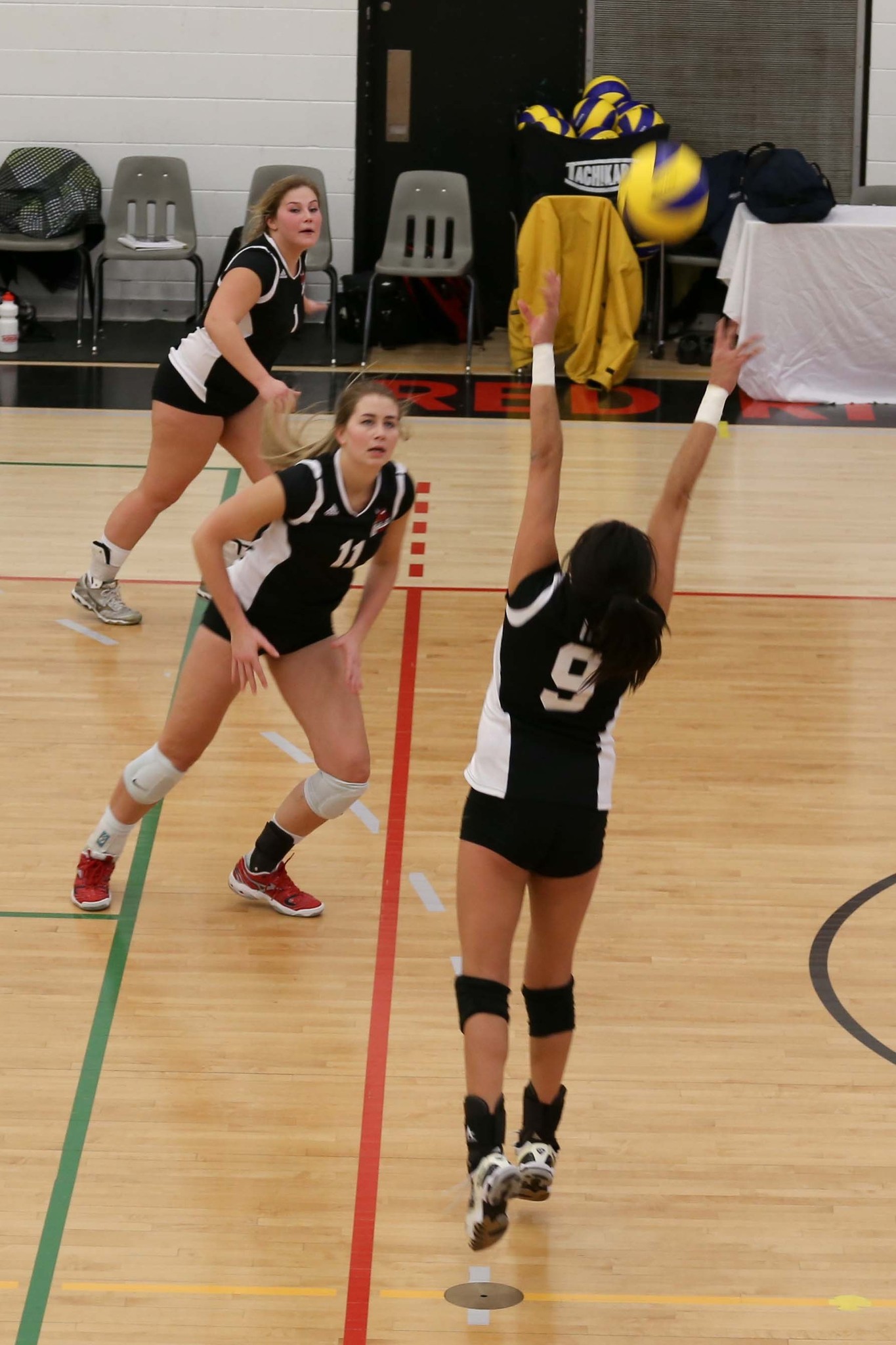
740 141 835 225
398 241 495 346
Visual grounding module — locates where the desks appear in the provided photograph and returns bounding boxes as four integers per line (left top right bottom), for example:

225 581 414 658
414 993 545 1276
718 202 896 404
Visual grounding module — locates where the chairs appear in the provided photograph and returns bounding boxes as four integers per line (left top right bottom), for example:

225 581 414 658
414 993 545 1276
0 145 206 355
358 167 482 372
236 162 342 369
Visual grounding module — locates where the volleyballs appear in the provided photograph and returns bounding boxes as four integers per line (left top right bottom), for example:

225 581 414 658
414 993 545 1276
573 98 619 135
517 104 565 131
532 115 575 138
617 101 650 122
579 126 619 140
617 107 663 134
617 137 709 243
583 74 631 108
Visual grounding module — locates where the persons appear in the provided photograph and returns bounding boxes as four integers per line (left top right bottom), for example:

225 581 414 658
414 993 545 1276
70 381 418 914
452 268 766 1251
73 174 330 624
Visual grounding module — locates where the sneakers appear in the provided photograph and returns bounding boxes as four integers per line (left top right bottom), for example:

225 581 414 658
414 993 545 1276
465 1152 522 1251
196 539 250 601
515 1136 561 1201
228 855 325 917
71 572 142 625
71 848 118 912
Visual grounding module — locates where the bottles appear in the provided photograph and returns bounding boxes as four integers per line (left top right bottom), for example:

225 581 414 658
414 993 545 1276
0 292 19 352
